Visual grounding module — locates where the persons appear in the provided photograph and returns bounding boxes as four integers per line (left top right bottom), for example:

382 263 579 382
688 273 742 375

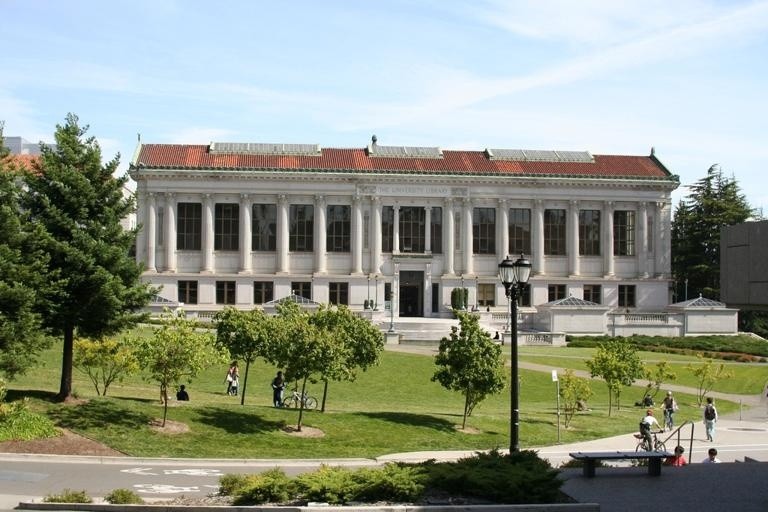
640 409 662 452
660 390 679 429
176 385 189 401
493 331 499 339
703 398 717 442
224 361 240 396
644 395 653 406
703 448 721 463
664 446 686 466
271 371 286 407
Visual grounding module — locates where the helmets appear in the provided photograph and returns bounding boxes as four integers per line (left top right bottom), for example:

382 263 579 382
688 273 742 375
667 391 671 395
647 409 653 413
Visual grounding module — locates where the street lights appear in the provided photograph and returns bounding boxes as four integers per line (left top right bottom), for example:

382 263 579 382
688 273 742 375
388 291 396 333
461 275 479 313
498 253 532 452
366 273 380 311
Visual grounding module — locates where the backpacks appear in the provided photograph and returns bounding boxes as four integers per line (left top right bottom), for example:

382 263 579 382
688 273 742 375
705 406 715 420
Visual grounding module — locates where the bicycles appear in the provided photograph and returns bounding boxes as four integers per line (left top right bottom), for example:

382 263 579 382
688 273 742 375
282 388 318 410
633 430 667 454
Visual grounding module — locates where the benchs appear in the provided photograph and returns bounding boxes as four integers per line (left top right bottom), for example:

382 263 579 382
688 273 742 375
568 450 675 477
